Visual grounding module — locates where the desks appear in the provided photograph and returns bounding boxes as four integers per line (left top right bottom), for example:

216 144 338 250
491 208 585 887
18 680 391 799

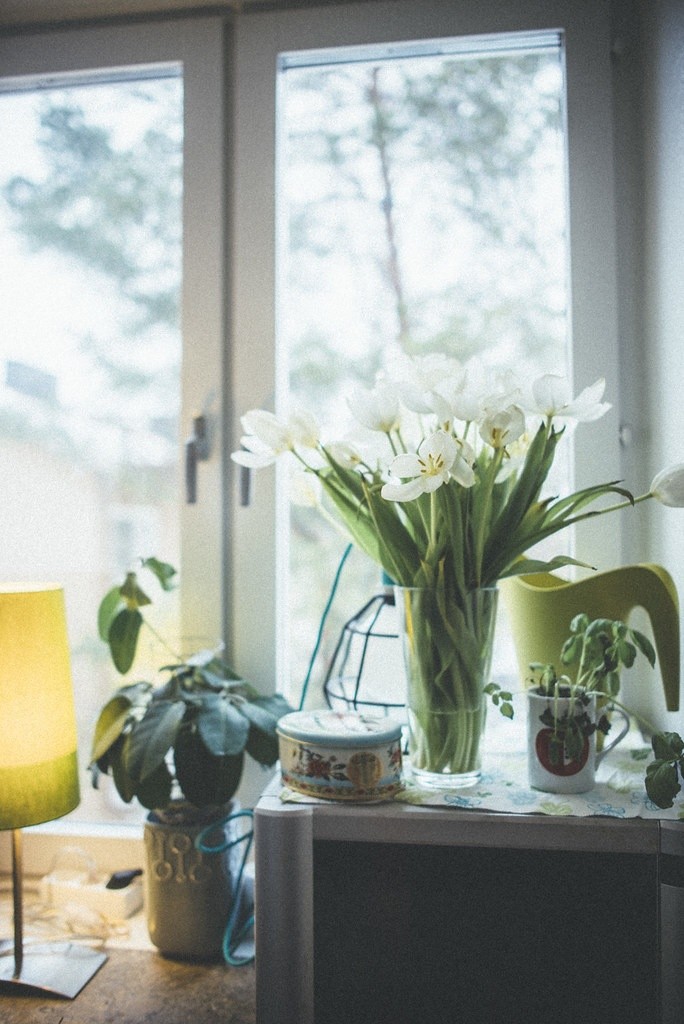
0 869 255 1024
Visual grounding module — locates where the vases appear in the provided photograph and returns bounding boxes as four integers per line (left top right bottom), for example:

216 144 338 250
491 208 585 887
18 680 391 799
391 584 499 790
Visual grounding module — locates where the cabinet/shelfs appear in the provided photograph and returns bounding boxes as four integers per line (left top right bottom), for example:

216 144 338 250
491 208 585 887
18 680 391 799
255 745 684 1024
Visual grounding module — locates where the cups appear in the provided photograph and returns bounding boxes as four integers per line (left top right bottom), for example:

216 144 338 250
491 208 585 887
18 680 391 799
525 685 631 795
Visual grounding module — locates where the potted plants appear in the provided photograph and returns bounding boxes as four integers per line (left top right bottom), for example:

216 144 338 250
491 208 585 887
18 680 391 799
84 549 295 959
483 612 684 810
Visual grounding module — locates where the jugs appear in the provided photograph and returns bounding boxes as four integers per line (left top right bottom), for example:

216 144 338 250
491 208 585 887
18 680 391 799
512 553 680 746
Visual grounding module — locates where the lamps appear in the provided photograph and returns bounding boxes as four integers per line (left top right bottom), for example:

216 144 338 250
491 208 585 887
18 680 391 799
0 580 108 1001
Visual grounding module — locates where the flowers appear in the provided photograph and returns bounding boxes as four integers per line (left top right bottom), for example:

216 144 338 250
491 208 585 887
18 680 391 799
229 347 684 589
